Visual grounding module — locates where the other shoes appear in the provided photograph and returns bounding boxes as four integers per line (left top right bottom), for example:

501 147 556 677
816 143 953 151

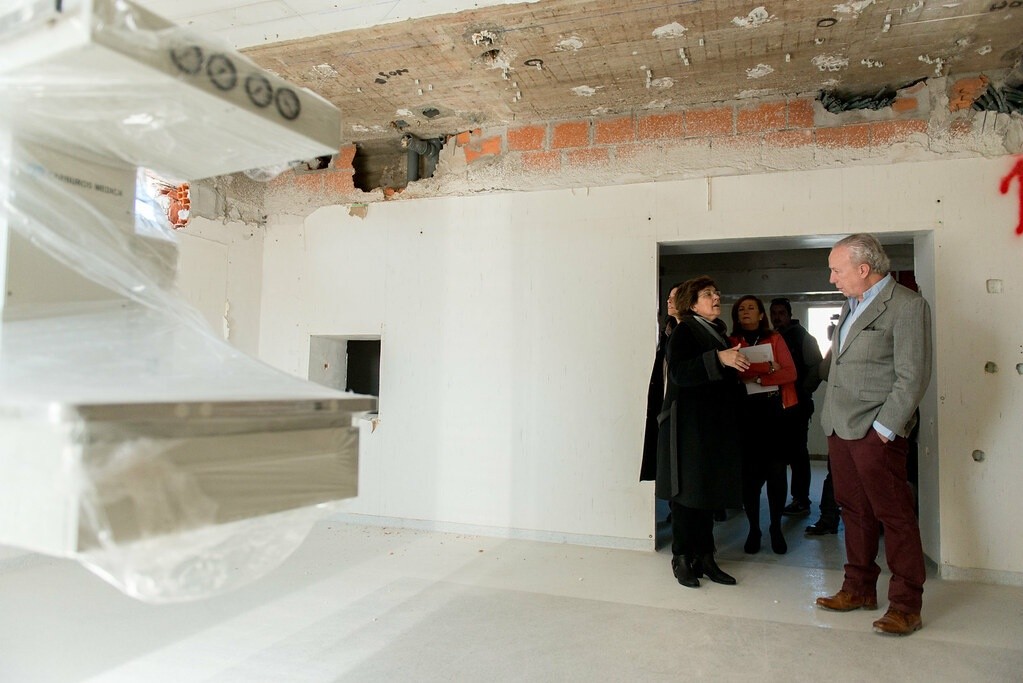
805 517 839 534
769 524 787 554
714 502 726 521
784 499 811 515
744 530 762 554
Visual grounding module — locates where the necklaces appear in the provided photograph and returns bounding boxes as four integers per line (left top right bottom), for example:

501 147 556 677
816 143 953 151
753 335 759 346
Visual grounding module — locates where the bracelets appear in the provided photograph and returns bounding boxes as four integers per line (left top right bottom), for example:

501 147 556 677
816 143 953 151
769 362 775 373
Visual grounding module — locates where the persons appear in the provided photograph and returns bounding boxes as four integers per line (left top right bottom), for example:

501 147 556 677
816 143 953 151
816 232 932 638
639 277 843 589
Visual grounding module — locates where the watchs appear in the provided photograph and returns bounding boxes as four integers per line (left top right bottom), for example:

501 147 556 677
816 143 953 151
756 376 762 384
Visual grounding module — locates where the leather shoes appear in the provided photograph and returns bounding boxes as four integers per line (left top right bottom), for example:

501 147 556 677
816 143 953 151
873 606 923 636
817 589 878 611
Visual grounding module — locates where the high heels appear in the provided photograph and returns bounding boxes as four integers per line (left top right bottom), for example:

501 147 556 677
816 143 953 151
690 554 736 584
672 554 700 587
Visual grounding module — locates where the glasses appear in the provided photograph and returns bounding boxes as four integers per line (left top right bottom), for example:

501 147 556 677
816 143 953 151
696 291 721 298
